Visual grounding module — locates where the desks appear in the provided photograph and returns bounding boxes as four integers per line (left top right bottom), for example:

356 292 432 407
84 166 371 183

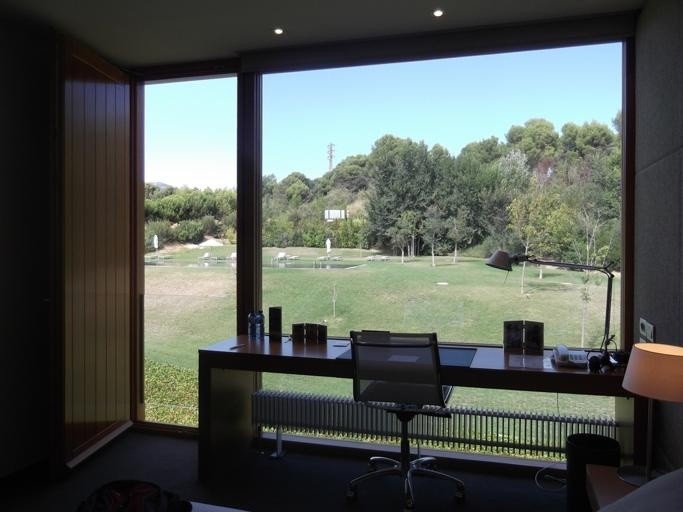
197 333 647 483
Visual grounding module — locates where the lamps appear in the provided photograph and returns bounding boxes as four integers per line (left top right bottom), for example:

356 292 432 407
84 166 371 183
615 341 683 486
484 250 613 365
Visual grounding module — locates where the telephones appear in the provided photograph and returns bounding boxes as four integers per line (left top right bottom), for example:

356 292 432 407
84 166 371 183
550 345 589 371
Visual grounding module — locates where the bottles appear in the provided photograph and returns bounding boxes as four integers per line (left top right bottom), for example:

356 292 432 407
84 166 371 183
256 310 264 339
247 309 256 338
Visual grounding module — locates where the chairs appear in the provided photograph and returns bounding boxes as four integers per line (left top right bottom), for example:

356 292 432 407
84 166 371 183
345 330 465 512
